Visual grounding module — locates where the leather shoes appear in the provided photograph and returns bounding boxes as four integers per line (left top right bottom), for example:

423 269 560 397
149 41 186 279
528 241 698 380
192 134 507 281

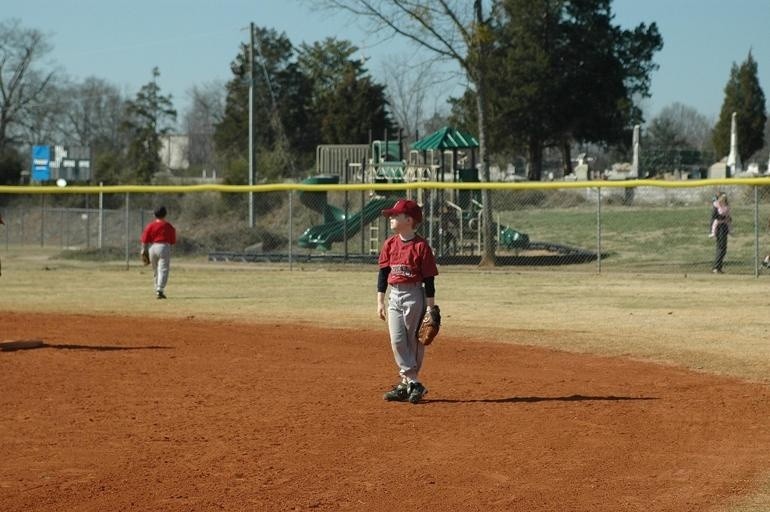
713 269 719 273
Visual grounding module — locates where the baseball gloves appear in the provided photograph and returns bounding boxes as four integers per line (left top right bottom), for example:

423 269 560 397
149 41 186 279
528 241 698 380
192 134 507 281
140 249 150 266
417 305 441 345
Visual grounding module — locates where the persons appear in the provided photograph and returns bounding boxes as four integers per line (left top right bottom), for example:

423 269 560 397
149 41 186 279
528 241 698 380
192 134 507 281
710 193 731 274
141 206 175 299
378 200 438 403
708 194 733 237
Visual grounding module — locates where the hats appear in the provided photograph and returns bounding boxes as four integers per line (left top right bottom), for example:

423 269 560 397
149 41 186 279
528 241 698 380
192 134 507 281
380 200 423 223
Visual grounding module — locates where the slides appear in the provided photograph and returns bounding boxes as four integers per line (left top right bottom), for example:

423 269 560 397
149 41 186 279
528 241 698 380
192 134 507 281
462 209 529 248
297 175 398 251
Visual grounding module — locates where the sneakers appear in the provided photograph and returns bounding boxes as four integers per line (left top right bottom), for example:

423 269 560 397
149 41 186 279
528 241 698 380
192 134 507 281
409 382 428 403
383 383 408 401
156 291 166 298
